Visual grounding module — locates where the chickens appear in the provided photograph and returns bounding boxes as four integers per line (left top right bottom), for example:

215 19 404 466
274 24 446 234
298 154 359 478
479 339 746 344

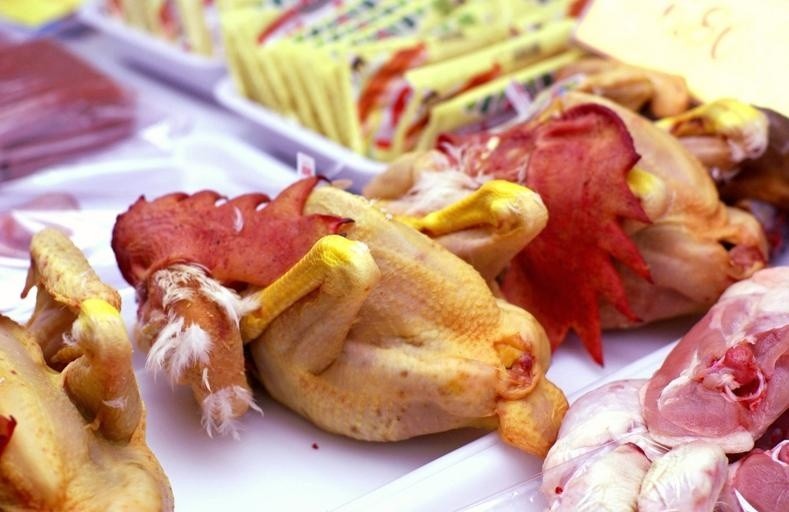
0 228 174 512
111 176 570 460
360 90 770 367
